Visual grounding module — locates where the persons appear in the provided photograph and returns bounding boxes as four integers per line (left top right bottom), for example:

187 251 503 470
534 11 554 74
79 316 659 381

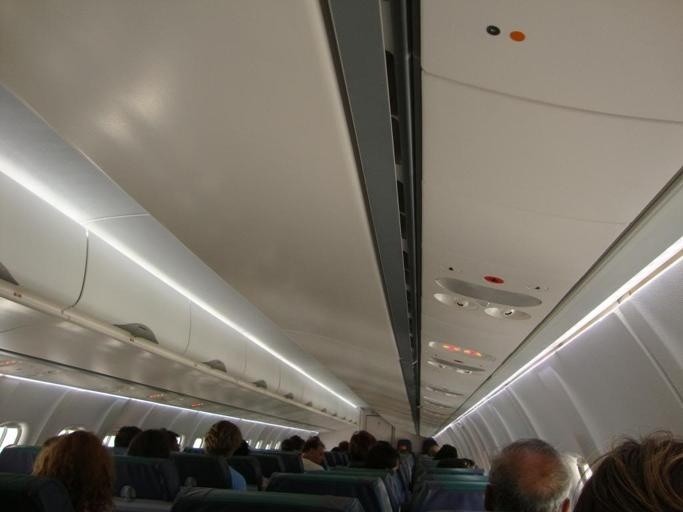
279 430 400 471
32 419 247 511
485 430 682 511
421 438 474 468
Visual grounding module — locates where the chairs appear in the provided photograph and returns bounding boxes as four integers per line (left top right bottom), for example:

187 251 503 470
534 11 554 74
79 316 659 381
1 442 499 512
